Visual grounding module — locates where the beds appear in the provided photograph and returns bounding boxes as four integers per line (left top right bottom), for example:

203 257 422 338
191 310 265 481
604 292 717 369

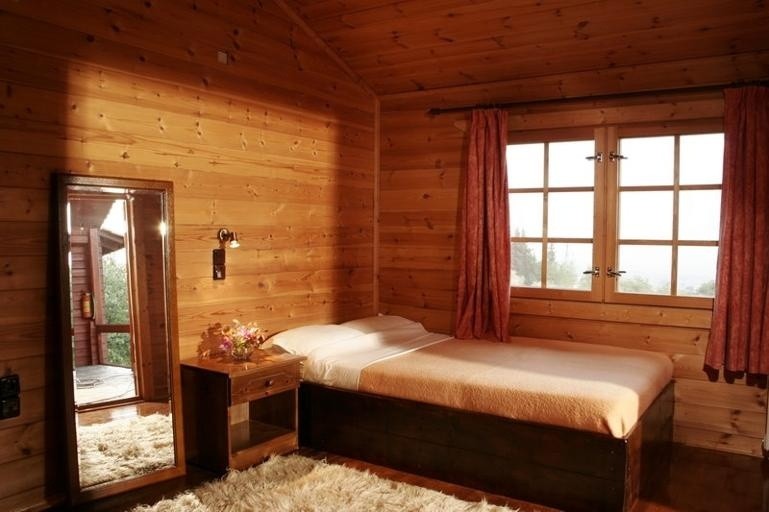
258 315 675 511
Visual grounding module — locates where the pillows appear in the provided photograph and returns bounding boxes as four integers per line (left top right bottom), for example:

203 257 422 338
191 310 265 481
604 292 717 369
259 324 365 361
342 314 426 343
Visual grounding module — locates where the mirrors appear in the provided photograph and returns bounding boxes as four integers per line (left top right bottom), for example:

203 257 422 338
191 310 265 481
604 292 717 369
54 173 187 505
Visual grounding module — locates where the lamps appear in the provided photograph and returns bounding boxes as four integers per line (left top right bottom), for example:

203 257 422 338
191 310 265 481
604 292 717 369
217 228 241 249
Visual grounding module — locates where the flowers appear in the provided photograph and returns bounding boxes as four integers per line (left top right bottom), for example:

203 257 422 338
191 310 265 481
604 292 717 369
196 319 267 363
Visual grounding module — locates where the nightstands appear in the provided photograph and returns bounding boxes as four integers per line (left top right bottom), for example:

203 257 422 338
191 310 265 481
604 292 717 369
180 349 307 472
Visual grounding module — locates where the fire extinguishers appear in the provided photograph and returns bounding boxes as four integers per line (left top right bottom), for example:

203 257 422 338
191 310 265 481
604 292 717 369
80 290 96 321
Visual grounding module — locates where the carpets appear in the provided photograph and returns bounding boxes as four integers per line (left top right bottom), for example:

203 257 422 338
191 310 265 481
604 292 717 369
75 413 175 489
130 452 519 512
